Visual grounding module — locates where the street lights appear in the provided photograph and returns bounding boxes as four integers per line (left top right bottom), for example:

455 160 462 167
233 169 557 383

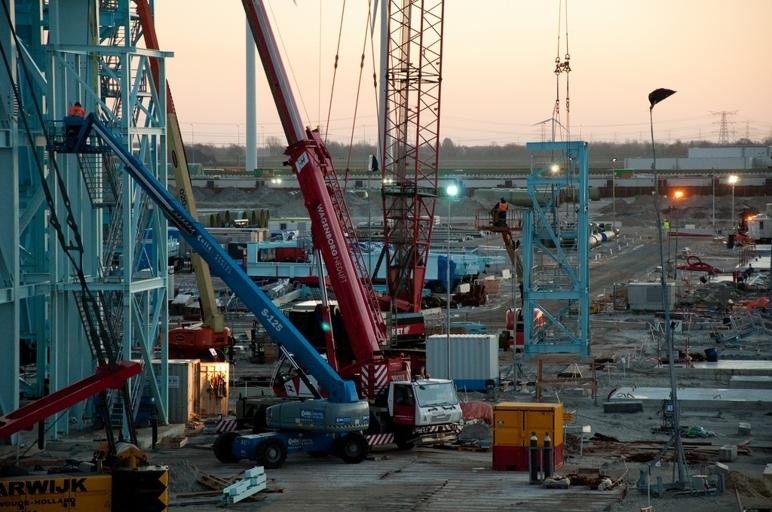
728 175 738 227
674 191 682 281
444 185 458 381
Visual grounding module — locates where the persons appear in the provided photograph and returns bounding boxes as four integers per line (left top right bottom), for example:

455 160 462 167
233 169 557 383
68 101 86 119
493 196 510 227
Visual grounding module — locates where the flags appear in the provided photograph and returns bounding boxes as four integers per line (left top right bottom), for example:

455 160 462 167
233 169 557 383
272 374 300 398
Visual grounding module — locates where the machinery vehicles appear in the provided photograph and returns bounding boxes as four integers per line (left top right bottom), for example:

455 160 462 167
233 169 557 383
133 0 237 366
241 0 466 451
0 360 170 512
45 111 371 470
474 208 546 353
